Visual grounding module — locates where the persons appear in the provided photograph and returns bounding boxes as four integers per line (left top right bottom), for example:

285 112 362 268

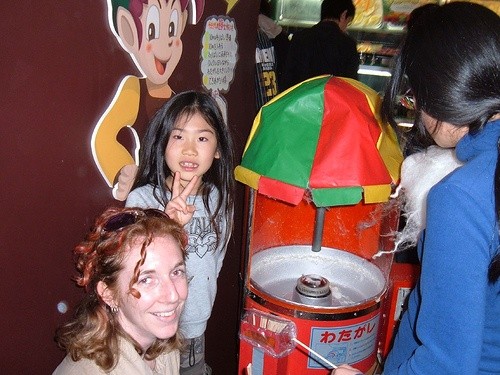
124 89 237 375
281 0 361 92
51 206 189 375
330 1 500 375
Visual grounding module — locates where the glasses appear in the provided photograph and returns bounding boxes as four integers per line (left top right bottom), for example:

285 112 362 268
399 86 415 111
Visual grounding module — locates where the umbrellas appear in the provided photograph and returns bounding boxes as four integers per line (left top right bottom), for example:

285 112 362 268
233 74 405 252
